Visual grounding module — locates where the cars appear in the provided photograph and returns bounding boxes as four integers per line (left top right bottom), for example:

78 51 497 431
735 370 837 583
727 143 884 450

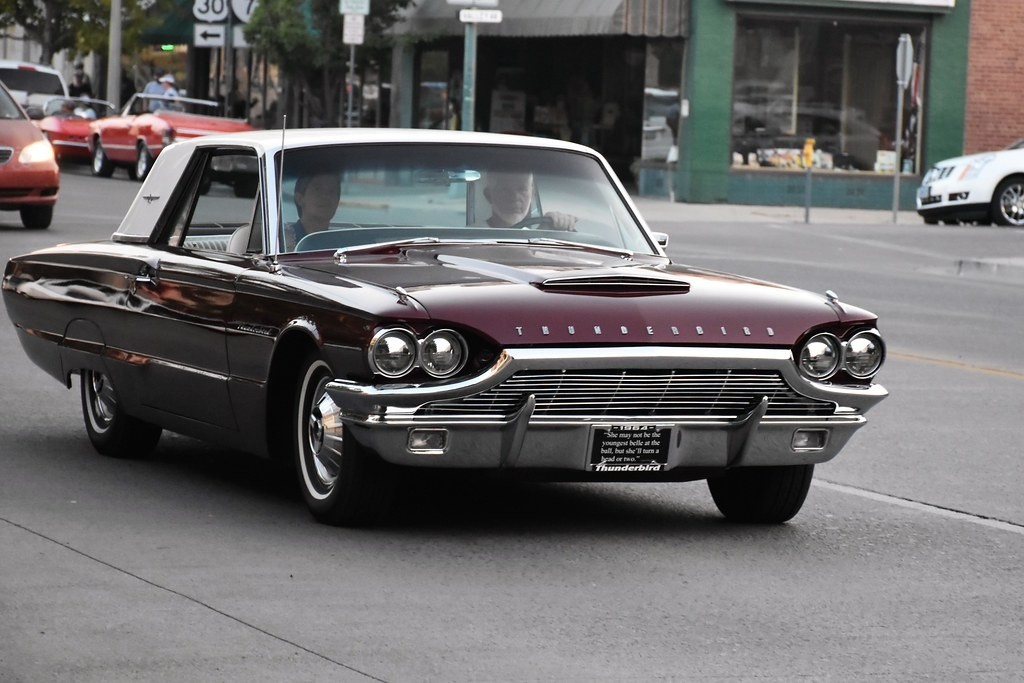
2 126 889 527
0 79 60 231
0 60 119 165
640 80 897 174
914 137 1024 230
87 91 268 181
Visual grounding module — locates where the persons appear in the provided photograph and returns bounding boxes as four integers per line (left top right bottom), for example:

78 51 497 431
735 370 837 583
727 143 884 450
465 153 579 231
230 80 247 118
52 61 181 119
280 165 341 253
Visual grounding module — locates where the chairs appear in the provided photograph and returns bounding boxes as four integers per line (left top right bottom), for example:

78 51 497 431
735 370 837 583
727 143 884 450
227 224 252 252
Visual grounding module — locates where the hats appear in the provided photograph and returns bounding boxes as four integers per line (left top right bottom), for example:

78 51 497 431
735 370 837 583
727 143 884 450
73 60 84 69
80 95 91 104
154 67 164 75
158 74 174 83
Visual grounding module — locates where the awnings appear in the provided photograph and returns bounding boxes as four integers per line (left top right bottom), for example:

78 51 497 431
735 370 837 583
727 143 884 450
412 0 689 40
